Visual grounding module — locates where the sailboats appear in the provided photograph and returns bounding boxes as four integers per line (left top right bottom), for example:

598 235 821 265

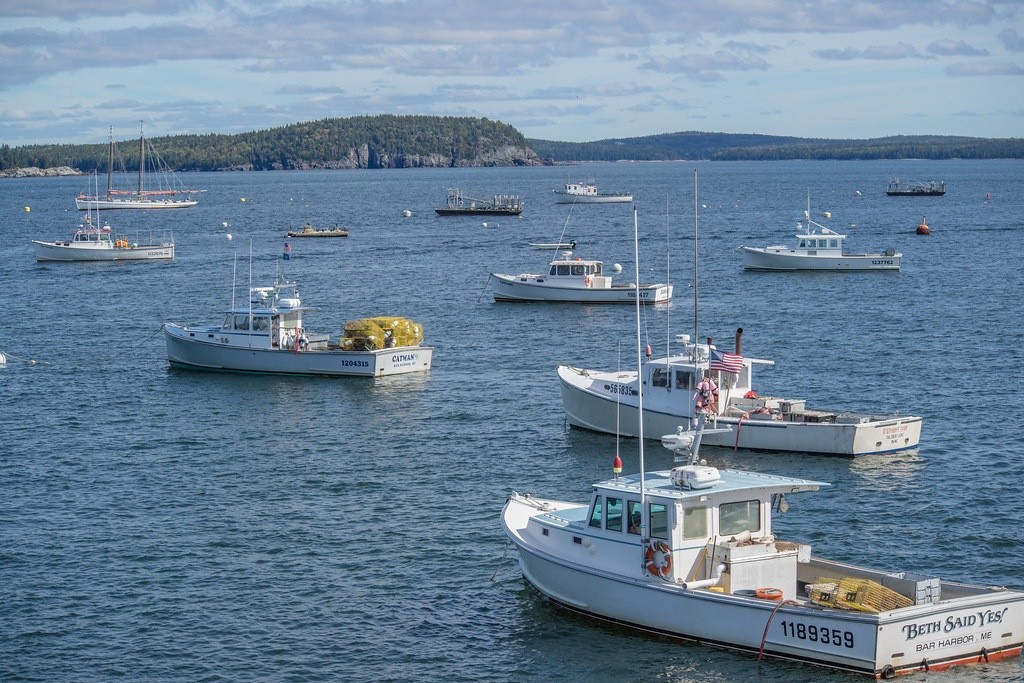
108 120 209 196
73 124 199 211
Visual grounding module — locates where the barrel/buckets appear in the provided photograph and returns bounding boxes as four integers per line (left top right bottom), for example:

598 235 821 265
733 589 756 597
756 587 784 600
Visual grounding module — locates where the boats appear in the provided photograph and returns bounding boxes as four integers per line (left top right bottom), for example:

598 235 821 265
434 187 525 217
554 204 924 455
485 239 675 305
161 235 435 377
524 241 579 252
497 204 1024 681
30 167 176 261
550 180 634 206
733 185 903 272
886 174 947 198
286 223 350 238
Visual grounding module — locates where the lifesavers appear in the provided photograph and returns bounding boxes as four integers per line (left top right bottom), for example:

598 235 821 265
585 276 590 286
645 541 672 576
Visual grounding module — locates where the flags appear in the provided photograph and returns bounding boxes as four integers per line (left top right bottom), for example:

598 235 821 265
283 253 290 260
284 243 291 251
710 349 743 373
